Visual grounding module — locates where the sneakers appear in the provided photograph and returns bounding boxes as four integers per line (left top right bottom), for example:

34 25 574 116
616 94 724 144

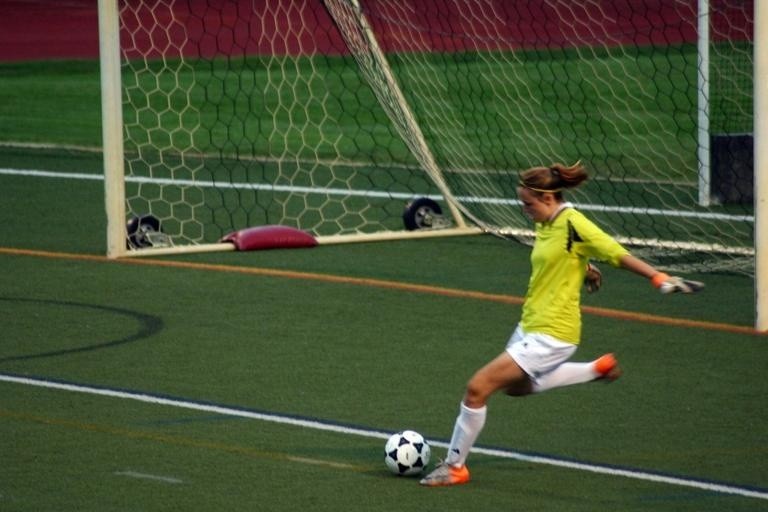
593 351 622 383
418 460 470 486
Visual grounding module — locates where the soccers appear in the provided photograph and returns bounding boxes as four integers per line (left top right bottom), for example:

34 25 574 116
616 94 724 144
384 430 430 475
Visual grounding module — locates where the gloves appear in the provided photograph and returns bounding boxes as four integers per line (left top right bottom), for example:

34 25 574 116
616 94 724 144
583 264 603 294
657 275 707 295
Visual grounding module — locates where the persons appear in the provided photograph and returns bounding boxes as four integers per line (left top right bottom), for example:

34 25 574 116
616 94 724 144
419 159 704 488
